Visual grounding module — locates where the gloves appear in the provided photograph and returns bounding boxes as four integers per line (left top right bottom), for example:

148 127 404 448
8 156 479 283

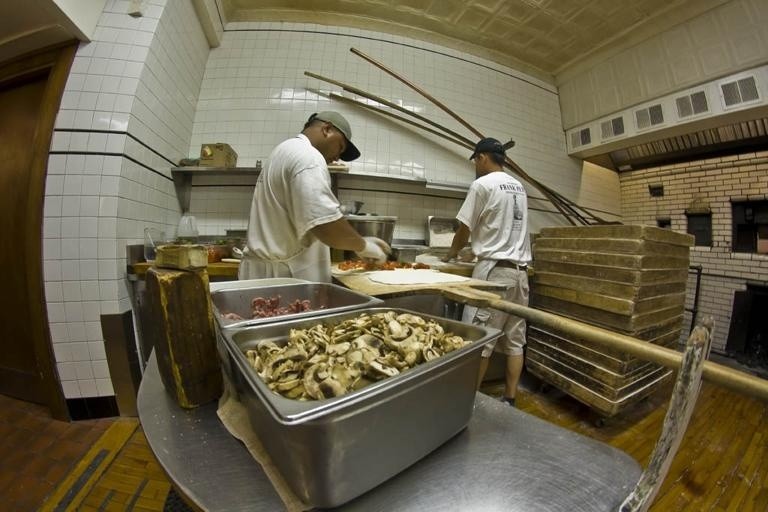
357 233 394 265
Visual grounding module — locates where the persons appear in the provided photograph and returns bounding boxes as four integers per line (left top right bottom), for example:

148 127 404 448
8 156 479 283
441 137 534 404
237 110 391 285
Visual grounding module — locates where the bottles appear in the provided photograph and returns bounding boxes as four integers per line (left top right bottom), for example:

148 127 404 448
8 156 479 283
256 160 262 168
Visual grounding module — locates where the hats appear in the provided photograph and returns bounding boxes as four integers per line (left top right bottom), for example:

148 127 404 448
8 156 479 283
467 136 505 161
308 110 362 162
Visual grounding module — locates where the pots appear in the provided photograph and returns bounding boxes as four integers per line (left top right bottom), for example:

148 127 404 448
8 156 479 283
344 212 395 260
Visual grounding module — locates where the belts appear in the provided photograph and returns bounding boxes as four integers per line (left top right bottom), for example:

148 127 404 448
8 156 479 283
493 259 528 272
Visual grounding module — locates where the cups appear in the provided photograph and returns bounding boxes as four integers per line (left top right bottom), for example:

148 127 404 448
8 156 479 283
143 227 165 264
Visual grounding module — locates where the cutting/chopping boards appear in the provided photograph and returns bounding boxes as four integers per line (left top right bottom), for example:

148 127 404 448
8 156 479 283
332 261 508 308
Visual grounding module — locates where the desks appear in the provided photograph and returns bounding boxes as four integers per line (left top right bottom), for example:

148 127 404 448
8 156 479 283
135 311 723 511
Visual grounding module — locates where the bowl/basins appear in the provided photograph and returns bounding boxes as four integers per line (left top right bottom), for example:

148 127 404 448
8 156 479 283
152 244 209 270
200 242 231 262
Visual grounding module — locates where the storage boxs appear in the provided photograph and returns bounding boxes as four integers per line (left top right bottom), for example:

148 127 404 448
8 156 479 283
199 142 240 168
